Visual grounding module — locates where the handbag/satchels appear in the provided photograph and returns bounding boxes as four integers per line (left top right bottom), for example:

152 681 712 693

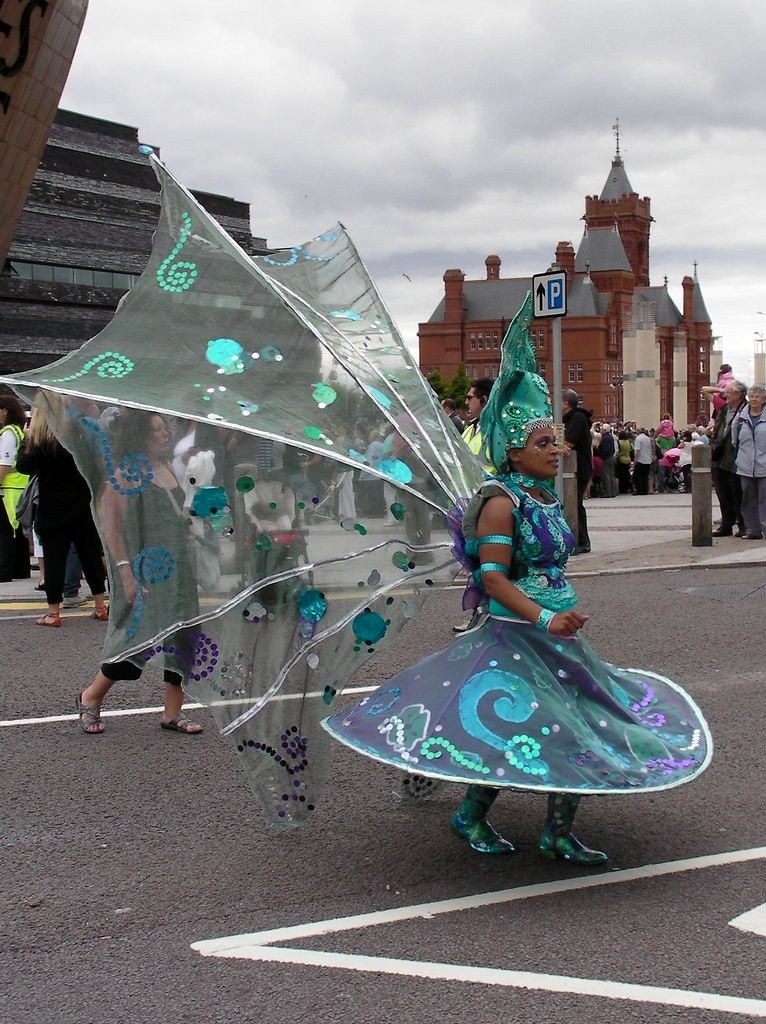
15 474 39 529
712 432 738 471
186 538 221 590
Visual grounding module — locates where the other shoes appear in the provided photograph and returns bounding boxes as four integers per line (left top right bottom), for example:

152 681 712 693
713 517 723 525
570 545 591 556
741 533 763 540
582 485 692 500
734 530 746 538
292 500 405 531
712 526 733 537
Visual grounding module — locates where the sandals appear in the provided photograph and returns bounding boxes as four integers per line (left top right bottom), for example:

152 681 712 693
35 612 61 626
35 578 46 591
91 605 109 621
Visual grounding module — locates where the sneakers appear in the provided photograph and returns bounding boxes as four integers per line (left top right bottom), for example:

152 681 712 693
62 595 88 608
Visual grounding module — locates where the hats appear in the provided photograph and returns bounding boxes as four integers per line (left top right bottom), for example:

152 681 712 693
480 290 554 475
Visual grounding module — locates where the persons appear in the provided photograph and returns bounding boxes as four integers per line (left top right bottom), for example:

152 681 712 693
0 365 766 627
319 292 712 864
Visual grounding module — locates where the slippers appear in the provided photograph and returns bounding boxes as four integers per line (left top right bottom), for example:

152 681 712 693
75 693 105 733
161 712 204 733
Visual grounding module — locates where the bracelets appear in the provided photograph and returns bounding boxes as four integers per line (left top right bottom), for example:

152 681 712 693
546 613 556 633
113 559 131 570
535 609 552 631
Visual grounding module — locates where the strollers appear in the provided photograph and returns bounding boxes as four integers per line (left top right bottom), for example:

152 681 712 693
304 471 347 525
663 463 693 494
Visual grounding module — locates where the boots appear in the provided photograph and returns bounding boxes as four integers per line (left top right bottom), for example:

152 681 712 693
450 784 515 853
539 791 609 865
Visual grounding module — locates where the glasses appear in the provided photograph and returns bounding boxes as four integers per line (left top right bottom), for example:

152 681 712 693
464 395 477 401
